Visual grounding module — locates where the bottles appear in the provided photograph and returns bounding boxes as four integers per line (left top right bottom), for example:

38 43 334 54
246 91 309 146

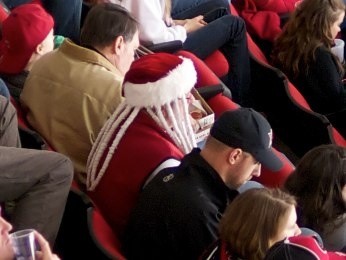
53 34 65 48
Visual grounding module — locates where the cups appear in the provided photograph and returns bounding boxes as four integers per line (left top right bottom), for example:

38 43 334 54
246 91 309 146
330 39 345 63
10 228 36 260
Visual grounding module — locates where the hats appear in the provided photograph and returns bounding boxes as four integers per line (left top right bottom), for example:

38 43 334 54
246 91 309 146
123 53 198 107
0 3 54 76
210 108 284 171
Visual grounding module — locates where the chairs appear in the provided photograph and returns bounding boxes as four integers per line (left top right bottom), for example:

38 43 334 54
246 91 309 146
8 1 346 260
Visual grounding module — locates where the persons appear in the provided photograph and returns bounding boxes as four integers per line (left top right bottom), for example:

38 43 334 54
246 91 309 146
0 0 346 260
